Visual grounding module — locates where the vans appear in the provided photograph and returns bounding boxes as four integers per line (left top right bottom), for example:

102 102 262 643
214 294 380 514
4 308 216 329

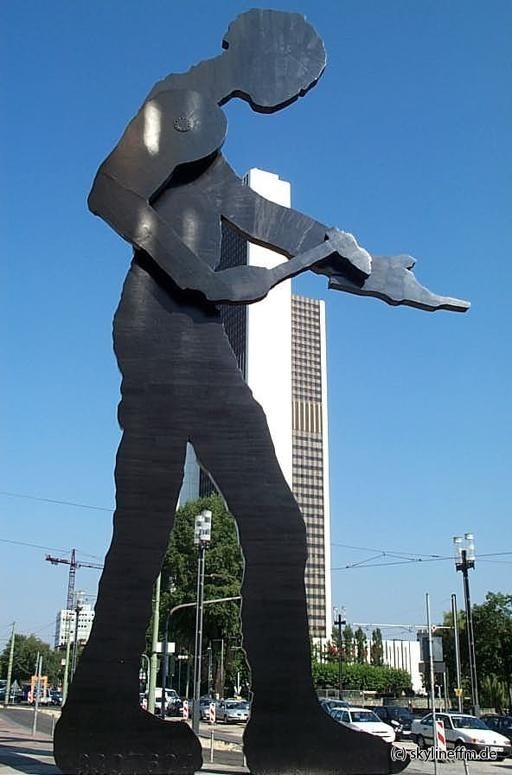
147 688 180 714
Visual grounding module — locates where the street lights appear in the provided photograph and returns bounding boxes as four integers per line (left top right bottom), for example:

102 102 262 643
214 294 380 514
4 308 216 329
192 510 212 731
333 606 347 696
452 532 479 711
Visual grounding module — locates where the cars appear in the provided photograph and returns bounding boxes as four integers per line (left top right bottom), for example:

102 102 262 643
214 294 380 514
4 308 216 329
167 697 250 725
409 713 512 761
0 680 63 705
321 699 414 744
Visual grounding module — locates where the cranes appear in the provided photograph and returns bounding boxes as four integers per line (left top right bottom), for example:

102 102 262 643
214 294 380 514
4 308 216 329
45 549 104 645
